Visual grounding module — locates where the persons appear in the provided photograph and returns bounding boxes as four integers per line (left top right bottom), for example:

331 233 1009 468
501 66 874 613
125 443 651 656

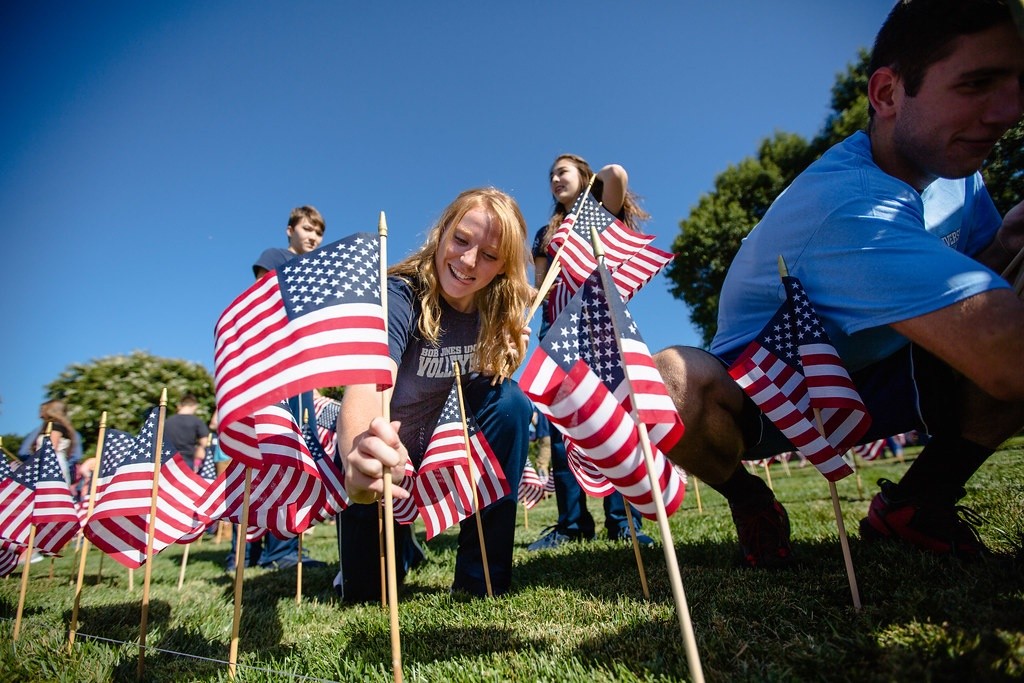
334 188 533 603
533 154 659 547
651 0 1024 565
19 401 85 506
162 395 210 472
32 401 75 461
879 436 903 458
223 207 328 573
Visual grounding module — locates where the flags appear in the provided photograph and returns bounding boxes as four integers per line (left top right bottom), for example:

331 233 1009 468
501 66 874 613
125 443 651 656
0 180 889 581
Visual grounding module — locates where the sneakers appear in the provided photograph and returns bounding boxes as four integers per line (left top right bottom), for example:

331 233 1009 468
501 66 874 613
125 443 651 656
729 492 795 574
860 478 993 566
607 525 654 548
528 525 576 552
223 553 258 573
263 547 327 572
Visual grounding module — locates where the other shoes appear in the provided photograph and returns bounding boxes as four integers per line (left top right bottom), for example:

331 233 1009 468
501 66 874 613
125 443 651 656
449 579 506 599
